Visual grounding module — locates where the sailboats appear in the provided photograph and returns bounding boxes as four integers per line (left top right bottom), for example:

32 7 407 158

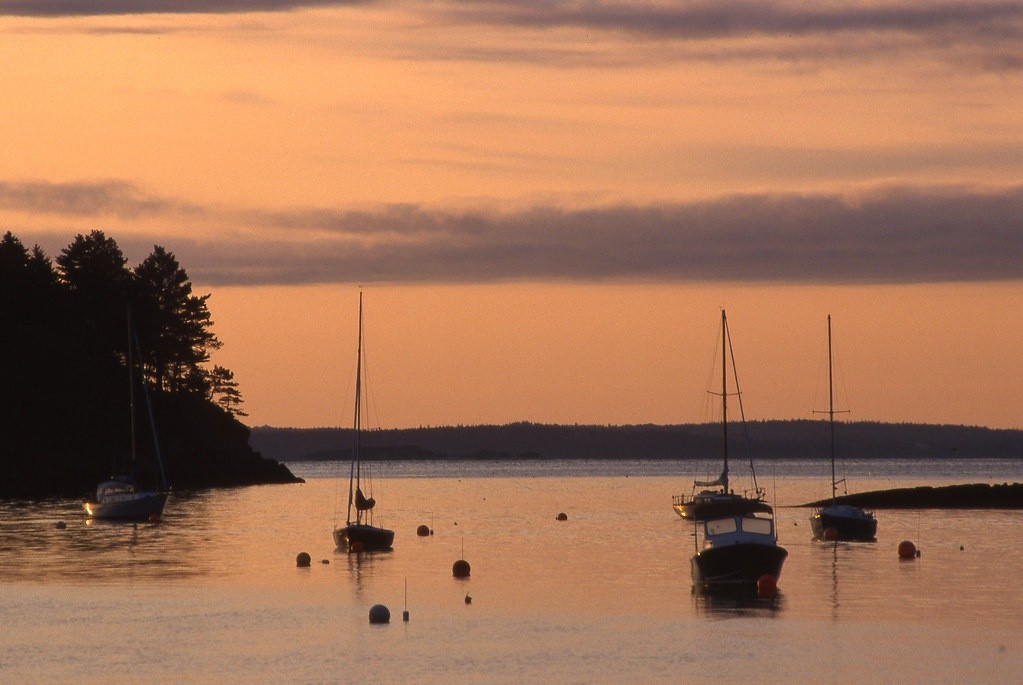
79 306 170 520
333 289 394 553
806 313 878 539
672 307 764 522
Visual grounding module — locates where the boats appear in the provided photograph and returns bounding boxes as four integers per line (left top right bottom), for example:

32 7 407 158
692 498 788 602
